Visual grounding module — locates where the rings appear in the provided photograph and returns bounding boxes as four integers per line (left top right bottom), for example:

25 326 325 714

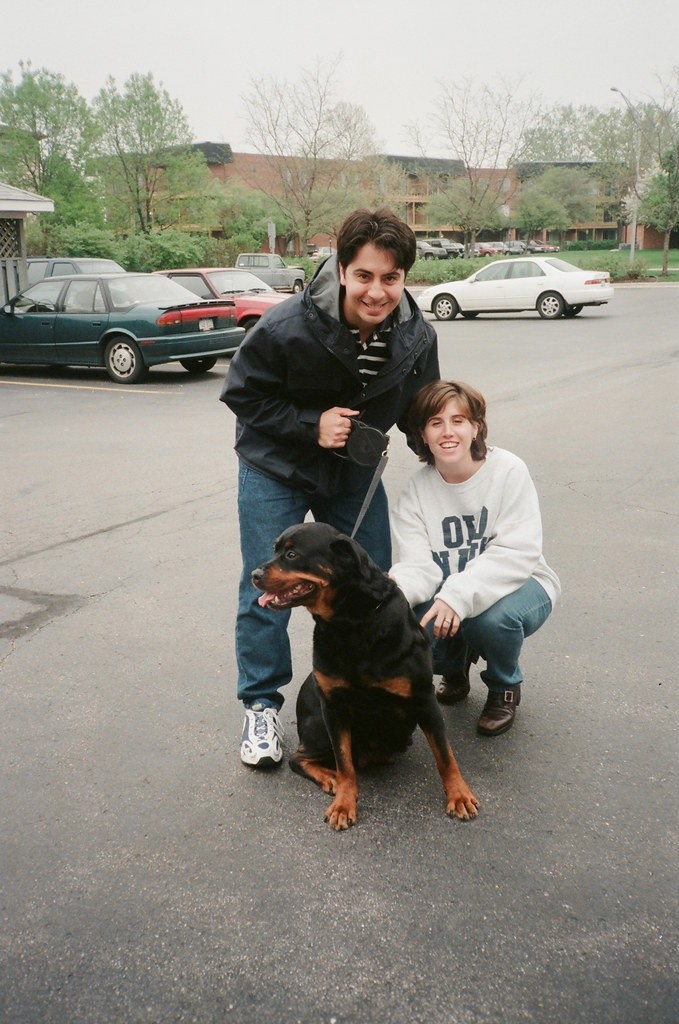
445 618 451 623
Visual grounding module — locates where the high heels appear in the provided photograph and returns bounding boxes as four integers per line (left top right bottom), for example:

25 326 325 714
436 650 479 702
478 685 521 736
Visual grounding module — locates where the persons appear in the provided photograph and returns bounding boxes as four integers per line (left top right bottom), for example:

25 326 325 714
387 378 562 736
219 207 441 768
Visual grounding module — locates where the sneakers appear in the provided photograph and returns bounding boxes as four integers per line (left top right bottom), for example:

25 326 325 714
240 708 285 768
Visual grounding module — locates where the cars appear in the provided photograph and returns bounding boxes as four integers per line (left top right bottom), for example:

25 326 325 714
1 273 246 384
415 256 612 321
150 267 295 357
415 240 447 260
452 239 560 258
423 239 459 259
18 258 127 300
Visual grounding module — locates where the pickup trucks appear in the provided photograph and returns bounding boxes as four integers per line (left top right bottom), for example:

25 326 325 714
230 253 307 296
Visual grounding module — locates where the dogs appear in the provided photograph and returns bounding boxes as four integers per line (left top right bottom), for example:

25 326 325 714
249 522 479 831
